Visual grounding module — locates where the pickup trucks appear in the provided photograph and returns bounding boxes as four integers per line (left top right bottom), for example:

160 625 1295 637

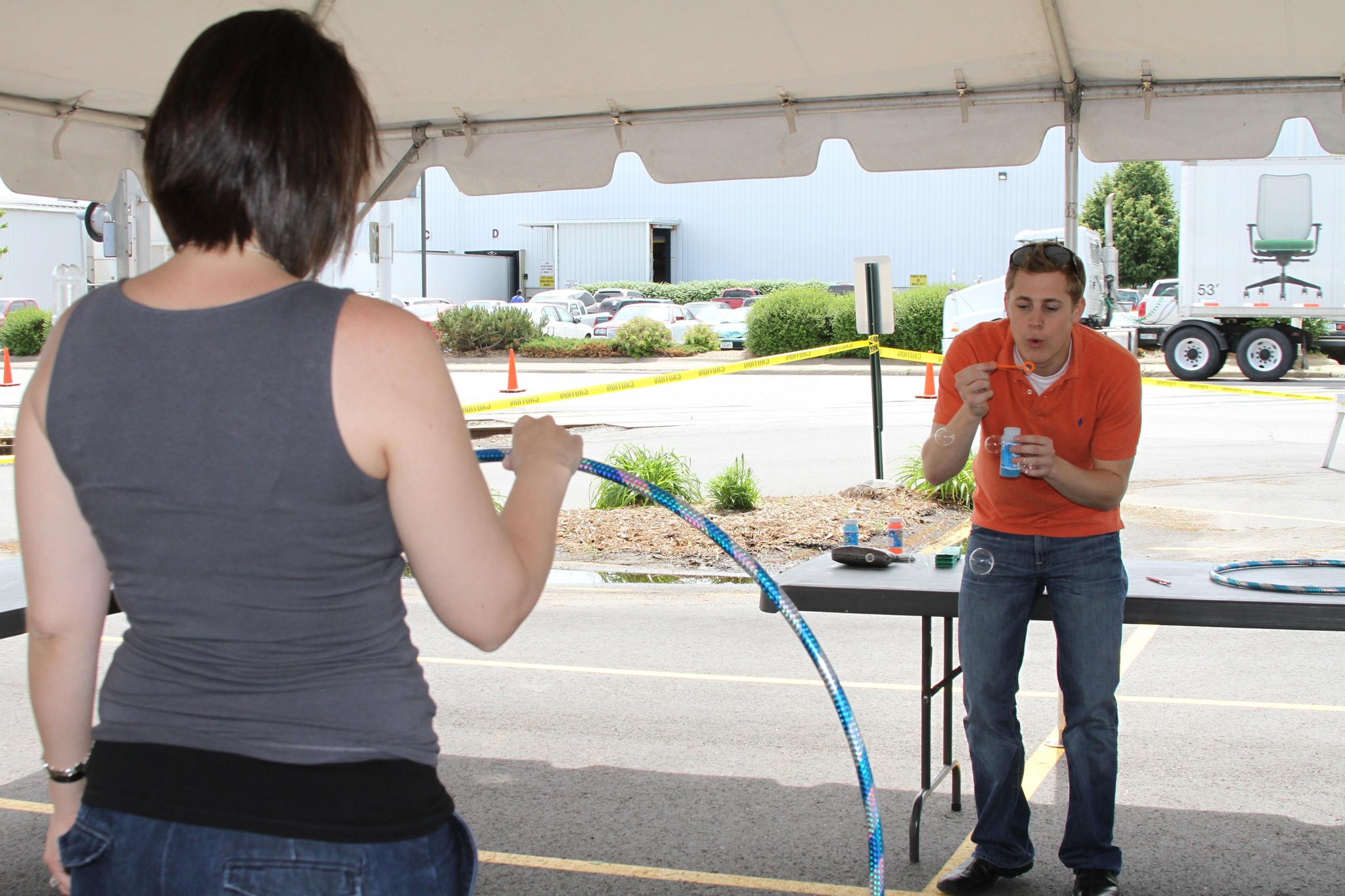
534 300 612 326
710 288 764 309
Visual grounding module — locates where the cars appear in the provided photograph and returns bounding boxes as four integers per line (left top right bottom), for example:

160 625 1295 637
356 291 463 341
680 301 734 321
528 289 599 313
616 298 675 316
1114 289 1146 311
741 295 765 308
0 297 42 325
597 295 635 316
497 303 594 340
593 303 707 349
711 306 758 352
462 299 509 317
592 288 648 302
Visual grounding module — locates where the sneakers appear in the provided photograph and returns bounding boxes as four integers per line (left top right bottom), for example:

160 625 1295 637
937 854 1035 896
1072 865 1119 896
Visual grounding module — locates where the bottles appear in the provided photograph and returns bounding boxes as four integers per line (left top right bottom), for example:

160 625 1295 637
888 517 903 554
1000 427 1021 477
843 519 859 546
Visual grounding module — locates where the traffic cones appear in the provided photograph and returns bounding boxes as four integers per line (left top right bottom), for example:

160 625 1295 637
497 347 525 392
0 347 28 388
912 350 943 398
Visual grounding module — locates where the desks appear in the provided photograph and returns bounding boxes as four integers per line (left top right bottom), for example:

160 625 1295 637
760 553 1345 864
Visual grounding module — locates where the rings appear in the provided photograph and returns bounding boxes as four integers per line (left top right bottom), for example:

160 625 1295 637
48 875 61 888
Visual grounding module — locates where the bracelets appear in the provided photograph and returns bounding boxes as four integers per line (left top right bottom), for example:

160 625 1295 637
41 742 95 784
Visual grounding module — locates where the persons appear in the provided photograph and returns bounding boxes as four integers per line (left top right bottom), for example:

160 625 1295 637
922 240 1140 896
14 8 583 896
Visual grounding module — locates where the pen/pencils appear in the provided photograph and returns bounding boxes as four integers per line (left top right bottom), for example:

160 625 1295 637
1146 576 1171 585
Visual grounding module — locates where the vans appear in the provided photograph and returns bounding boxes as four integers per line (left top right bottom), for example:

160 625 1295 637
828 283 855 296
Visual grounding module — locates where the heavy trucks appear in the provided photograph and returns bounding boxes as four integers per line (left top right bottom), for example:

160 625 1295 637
940 155 1345 384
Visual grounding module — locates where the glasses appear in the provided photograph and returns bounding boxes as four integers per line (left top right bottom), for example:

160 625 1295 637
1009 245 1082 284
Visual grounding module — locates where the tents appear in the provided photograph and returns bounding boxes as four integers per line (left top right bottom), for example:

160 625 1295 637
0 0 1345 294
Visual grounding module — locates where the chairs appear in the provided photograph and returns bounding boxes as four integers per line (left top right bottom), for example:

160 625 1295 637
1243 174 1323 301
